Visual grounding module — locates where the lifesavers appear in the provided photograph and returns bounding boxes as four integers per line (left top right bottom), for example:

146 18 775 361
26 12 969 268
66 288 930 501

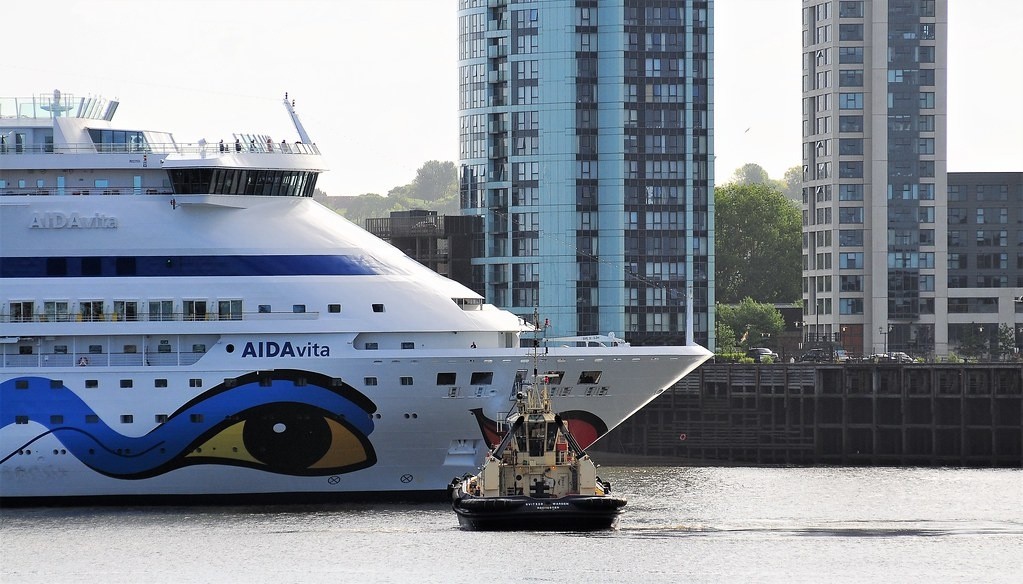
679 434 687 441
78 357 88 367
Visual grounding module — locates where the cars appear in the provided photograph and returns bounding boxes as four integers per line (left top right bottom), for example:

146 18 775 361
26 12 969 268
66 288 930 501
746 346 913 365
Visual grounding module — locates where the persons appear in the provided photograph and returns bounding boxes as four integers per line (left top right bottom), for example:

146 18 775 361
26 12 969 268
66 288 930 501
530 423 542 455
234 139 242 151
249 140 257 152
280 139 288 152
266 138 275 152
470 341 476 348
219 139 230 153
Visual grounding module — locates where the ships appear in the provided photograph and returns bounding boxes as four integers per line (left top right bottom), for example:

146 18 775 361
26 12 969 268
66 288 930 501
0 88 719 511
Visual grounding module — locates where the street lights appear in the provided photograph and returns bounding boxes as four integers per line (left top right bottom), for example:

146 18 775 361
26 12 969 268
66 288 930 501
794 321 806 352
977 324 984 361
745 323 752 359
877 326 893 355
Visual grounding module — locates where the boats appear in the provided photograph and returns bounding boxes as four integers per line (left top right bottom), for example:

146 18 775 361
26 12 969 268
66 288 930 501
447 305 627 535
